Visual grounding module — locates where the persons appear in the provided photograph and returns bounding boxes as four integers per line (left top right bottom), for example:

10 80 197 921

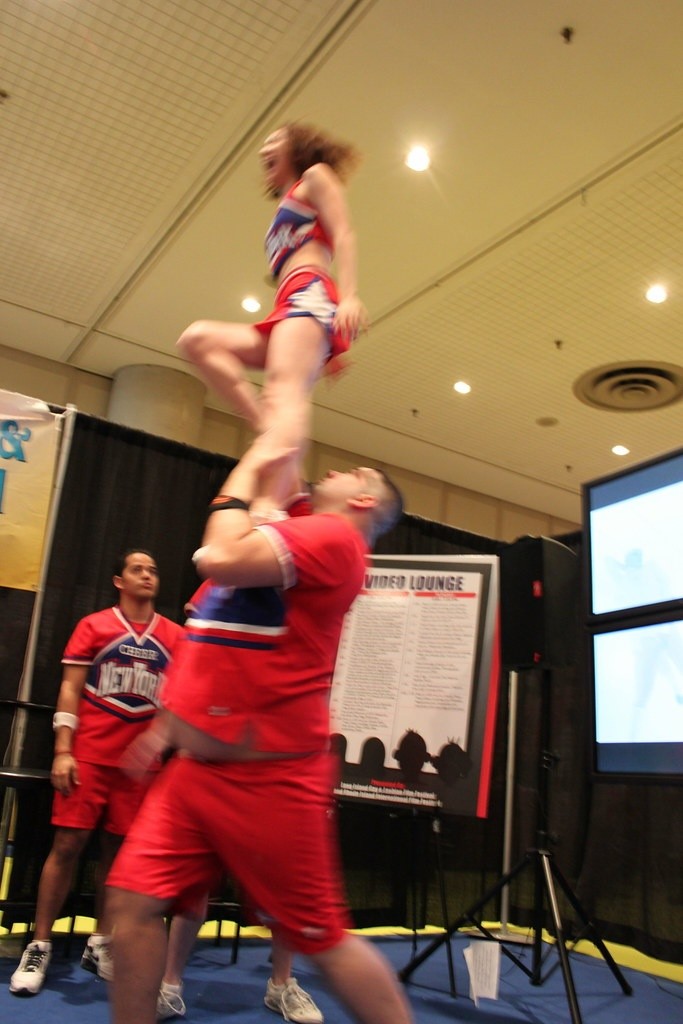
176 122 359 510
104 466 413 1024
156 885 323 1024
8 548 181 994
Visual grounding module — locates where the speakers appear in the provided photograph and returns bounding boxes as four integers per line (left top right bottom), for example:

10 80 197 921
501 534 578 670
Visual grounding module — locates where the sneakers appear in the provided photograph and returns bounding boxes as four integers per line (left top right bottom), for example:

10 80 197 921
79 933 116 983
9 939 52 995
154 989 188 1022
264 976 326 1024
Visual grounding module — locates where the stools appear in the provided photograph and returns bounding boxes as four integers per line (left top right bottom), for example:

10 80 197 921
0 767 77 948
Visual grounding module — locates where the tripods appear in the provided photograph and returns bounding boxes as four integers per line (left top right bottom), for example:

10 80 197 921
396 669 633 1024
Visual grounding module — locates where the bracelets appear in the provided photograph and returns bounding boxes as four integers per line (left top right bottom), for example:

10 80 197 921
54 747 71 753
208 496 249 511
52 712 79 731
